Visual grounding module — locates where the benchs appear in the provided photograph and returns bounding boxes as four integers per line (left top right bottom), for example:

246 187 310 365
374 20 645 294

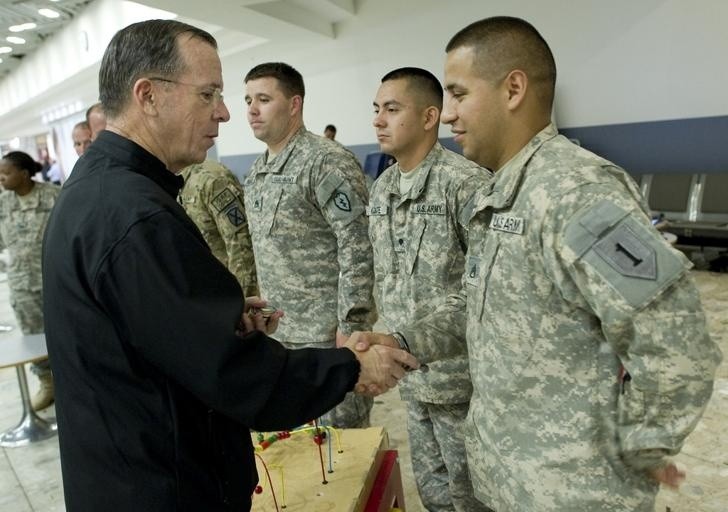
628 171 728 257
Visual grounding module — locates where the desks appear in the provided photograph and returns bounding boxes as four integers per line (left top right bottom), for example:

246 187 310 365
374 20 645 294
0 334 57 447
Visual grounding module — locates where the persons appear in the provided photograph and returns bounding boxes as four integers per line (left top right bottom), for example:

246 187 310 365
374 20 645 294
240 62 374 429
351 16 721 510
366 67 495 511
322 125 336 140
177 161 260 304
0 101 113 412
40 20 420 512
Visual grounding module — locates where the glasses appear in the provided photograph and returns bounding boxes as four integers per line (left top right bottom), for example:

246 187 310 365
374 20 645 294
150 77 221 105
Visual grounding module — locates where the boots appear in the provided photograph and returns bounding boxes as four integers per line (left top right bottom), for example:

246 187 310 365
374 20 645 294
31 374 54 410
